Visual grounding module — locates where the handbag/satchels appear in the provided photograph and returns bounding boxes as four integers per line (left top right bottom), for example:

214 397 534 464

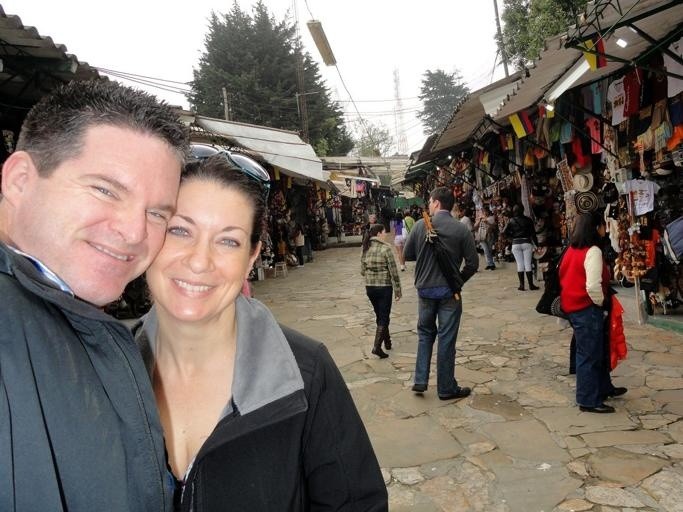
293 231 306 248
401 218 409 241
534 267 573 322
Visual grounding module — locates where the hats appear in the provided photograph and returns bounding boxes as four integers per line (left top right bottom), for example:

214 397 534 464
533 246 547 261
572 171 596 193
575 190 600 215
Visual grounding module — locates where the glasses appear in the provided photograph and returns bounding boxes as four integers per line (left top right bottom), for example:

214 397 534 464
185 143 272 211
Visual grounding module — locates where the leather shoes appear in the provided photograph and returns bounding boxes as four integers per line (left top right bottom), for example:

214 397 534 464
579 403 615 414
609 386 628 397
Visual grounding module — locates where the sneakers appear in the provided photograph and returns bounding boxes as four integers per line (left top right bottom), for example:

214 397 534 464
439 384 471 401
400 264 408 272
411 383 428 392
485 265 496 270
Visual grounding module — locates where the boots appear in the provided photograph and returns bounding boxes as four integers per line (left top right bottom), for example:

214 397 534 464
517 271 525 291
382 327 392 352
525 270 540 290
370 325 390 359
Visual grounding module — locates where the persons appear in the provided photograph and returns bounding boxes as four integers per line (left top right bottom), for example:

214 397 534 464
1 79 187 512
128 144 388 511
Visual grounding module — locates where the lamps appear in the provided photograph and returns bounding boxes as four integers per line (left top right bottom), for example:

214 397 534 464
305 19 336 69
609 33 627 49
542 55 592 107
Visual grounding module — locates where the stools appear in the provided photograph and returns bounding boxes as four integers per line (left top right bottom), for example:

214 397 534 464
273 261 287 279
